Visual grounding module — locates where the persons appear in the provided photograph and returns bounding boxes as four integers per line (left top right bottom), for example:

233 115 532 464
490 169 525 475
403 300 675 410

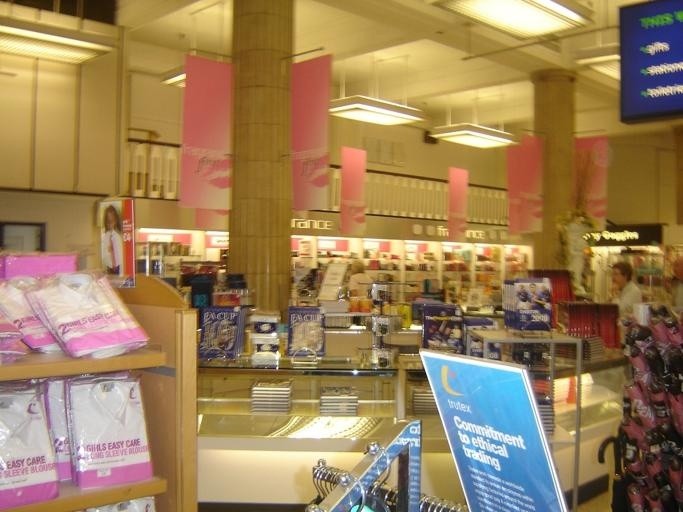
99 204 121 276
609 262 644 343
515 284 529 310
526 283 546 310
345 261 373 298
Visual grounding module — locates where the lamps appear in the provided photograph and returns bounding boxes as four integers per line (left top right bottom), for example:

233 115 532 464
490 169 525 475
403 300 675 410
0 0 185 90
